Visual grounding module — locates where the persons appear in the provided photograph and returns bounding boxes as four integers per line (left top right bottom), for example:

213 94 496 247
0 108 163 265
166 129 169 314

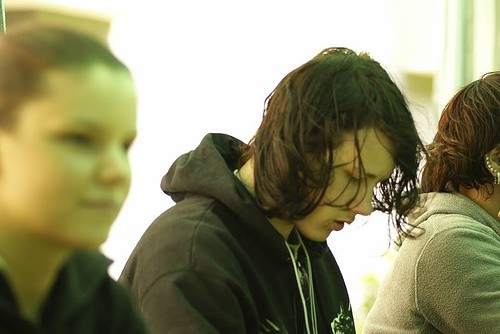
117 47 432 334
362 71 500 334
0 24 152 334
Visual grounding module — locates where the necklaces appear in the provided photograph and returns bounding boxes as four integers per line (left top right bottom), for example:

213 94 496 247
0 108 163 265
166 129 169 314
286 226 319 334
